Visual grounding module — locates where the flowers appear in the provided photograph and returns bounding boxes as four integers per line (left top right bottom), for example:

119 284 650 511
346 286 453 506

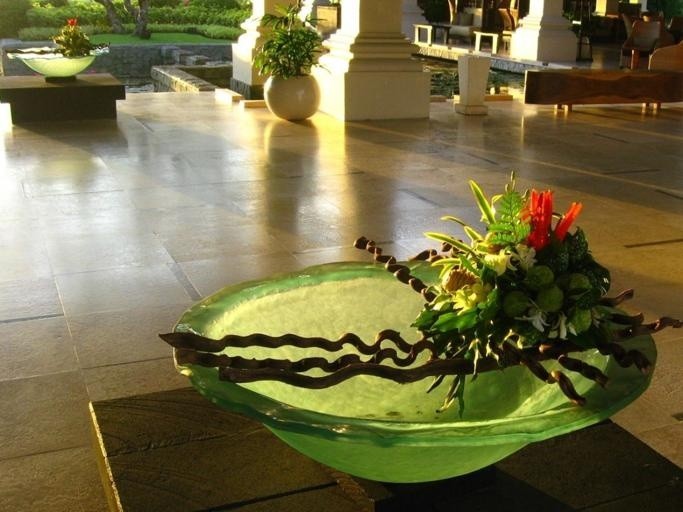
408 169 615 417
51 18 90 56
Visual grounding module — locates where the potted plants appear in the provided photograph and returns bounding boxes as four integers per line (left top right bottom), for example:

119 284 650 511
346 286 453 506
254 0 321 120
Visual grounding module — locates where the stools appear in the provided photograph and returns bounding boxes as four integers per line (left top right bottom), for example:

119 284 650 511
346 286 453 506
472 29 511 55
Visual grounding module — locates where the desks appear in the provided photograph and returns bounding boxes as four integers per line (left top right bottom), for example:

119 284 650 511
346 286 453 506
413 22 452 44
0 73 126 123
90 387 683 512
